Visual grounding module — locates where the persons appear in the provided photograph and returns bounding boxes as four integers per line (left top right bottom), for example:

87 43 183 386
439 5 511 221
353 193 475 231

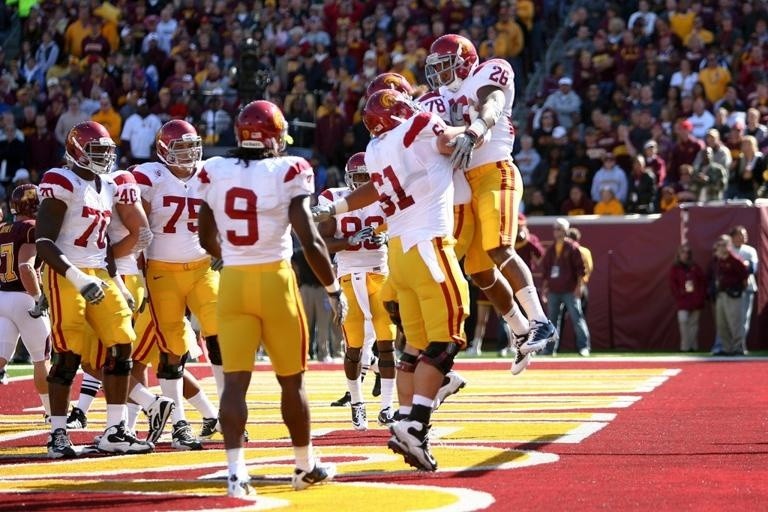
673 226 760 356
458 216 595 357
2 1 767 243
201 98 348 501
0 120 404 459
361 93 490 471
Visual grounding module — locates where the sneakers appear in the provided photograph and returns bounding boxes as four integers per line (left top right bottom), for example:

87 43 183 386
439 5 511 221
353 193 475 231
292 459 336 491
519 320 559 354
227 475 256 499
512 331 531 375
43 394 249 458
580 349 590 357
331 369 466 471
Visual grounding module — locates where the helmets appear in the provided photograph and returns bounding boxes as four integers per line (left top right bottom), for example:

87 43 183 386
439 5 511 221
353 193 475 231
157 120 203 168
10 184 39 220
64 122 116 173
234 101 289 152
344 152 370 191
362 35 480 137
